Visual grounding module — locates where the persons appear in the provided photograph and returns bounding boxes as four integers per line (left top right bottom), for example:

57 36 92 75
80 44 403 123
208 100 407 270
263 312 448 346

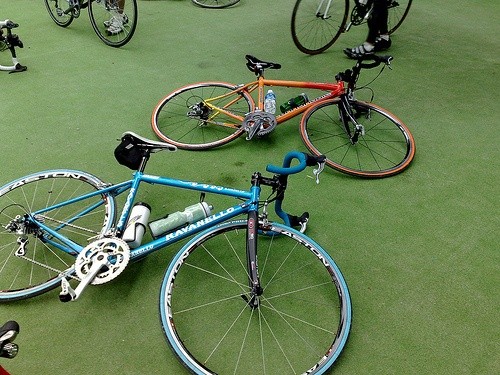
343 0 392 57
104 0 128 35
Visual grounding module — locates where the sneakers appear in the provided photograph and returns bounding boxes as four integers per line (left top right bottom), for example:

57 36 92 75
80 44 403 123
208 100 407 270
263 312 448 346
104 13 129 27
374 38 392 50
105 21 124 36
343 43 376 58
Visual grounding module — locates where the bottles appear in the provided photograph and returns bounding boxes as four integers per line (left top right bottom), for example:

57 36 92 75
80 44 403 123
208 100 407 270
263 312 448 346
264 89 276 114
147 203 214 239
123 202 153 249
358 0 368 5
279 93 308 112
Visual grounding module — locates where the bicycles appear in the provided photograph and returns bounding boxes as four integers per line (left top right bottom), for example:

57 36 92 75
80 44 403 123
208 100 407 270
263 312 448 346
192 0 241 9
150 52 416 179
0 132 351 375
44 0 138 48
0 19 28 75
290 0 415 54
